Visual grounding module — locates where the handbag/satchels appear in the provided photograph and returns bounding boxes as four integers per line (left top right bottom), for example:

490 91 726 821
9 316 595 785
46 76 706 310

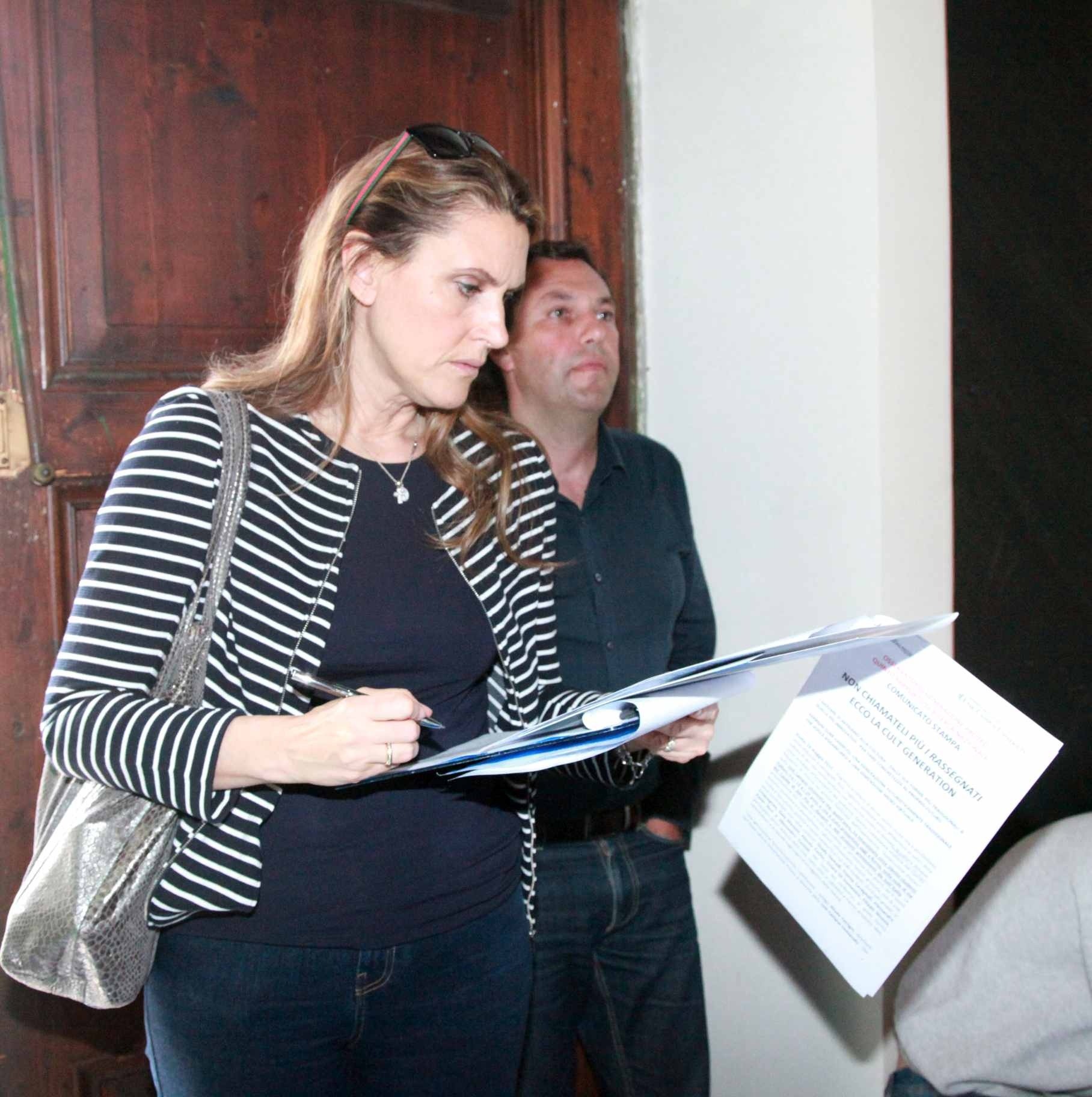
0 388 251 1008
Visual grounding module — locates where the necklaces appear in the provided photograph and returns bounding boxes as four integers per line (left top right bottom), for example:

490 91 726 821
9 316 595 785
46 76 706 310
329 400 420 504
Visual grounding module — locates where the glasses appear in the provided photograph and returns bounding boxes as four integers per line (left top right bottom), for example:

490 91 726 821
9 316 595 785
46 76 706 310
344 124 518 229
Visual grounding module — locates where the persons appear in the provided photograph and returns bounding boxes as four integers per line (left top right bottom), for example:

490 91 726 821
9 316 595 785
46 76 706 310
39 122 717 1097
493 243 715 1097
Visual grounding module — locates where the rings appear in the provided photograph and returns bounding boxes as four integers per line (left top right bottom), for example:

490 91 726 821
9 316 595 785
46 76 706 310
386 742 397 766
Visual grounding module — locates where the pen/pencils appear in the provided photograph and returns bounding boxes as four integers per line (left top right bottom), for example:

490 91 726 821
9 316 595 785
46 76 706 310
289 669 446 731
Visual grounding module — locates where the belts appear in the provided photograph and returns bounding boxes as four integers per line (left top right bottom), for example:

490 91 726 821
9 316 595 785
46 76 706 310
536 801 640 844
